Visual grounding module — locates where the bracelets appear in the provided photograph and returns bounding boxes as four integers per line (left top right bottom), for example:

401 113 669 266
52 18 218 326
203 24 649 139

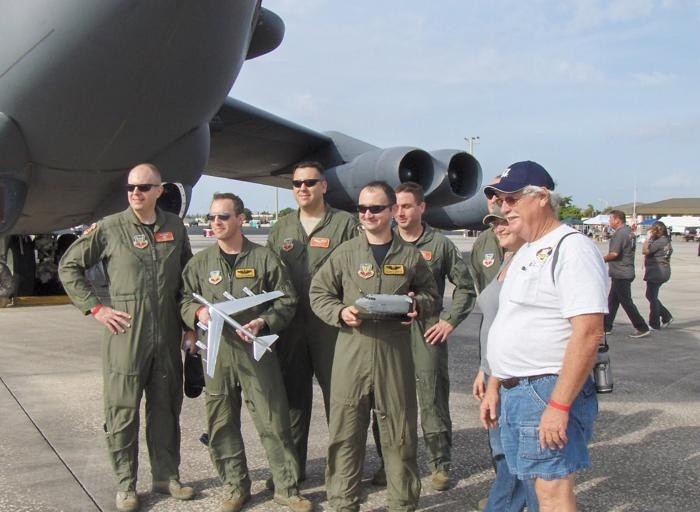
195 306 205 319
548 398 573 412
90 304 104 316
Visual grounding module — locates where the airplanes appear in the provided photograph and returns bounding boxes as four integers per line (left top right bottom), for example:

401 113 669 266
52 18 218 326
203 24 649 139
181 286 286 379
354 291 413 319
0 0 490 309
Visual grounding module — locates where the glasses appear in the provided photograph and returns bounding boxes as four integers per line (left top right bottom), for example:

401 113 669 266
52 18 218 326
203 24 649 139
358 205 390 213
496 191 531 206
292 179 320 187
208 212 230 219
126 184 159 191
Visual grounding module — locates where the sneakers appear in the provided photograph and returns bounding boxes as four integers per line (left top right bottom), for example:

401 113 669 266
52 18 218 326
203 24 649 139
373 464 386 485
153 479 194 499
266 473 312 512
116 491 139 512
224 489 250 512
431 469 449 490
629 317 673 338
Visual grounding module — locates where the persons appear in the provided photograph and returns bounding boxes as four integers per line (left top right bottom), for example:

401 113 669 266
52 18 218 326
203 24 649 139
600 210 652 339
180 192 315 511
59 162 198 510
260 159 365 489
308 178 444 511
469 200 529 512
469 175 530 291
0 261 17 296
585 225 610 242
640 221 674 329
368 181 479 493
472 159 612 511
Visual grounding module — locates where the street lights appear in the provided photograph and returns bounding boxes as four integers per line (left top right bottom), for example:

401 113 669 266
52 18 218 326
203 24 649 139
464 136 480 154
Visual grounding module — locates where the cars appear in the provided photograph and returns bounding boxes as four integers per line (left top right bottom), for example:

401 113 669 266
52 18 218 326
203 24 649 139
191 220 199 227
184 222 190 227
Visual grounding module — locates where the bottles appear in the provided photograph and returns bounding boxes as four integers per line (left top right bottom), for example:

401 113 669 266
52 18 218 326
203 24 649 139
593 342 614 396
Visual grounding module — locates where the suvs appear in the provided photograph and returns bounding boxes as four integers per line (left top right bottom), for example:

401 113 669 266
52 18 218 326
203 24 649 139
684 226 700 242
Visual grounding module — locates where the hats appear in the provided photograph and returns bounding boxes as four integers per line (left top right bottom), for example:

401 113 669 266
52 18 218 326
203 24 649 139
482 161 554 225
185 348 205 397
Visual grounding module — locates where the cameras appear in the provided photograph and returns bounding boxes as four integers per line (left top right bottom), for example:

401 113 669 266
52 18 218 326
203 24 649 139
592 346 613 393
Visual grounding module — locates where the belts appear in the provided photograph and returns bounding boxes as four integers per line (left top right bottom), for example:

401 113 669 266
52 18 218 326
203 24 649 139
501 374 554 388
